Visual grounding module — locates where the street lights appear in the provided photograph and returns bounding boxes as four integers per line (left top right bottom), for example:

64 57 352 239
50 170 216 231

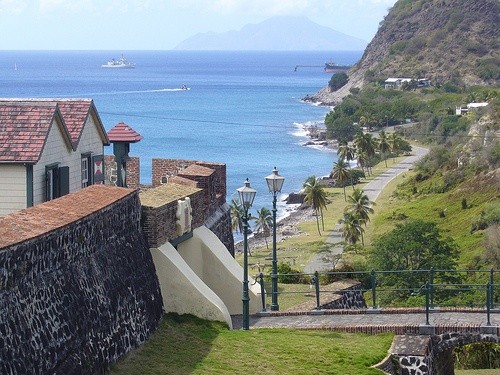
265 166 285 310
236 177 257 331
